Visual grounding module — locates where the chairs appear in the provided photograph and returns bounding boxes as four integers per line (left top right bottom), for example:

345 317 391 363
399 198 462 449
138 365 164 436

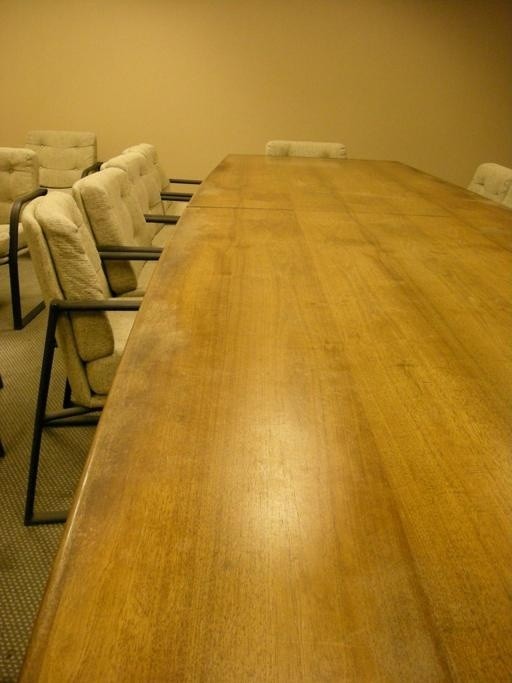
263 133 349 159
469 163 512 200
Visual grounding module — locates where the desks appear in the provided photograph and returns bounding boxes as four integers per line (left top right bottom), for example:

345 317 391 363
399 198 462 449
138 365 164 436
17 123 511 681
